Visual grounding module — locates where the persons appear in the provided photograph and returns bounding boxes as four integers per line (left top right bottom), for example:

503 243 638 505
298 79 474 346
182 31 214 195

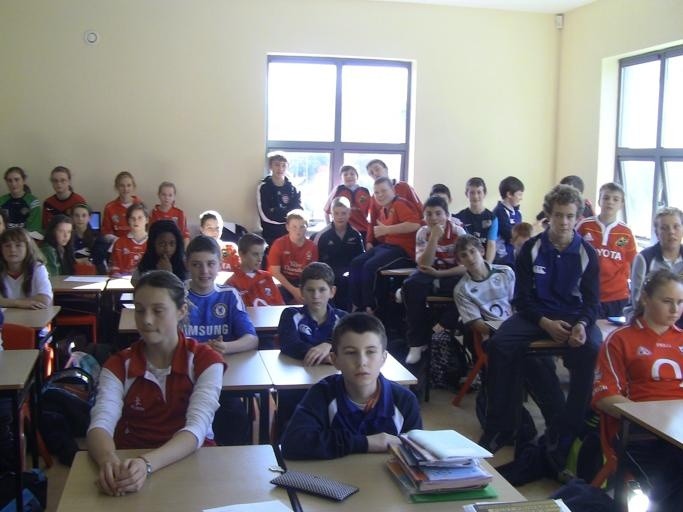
1 154 682 510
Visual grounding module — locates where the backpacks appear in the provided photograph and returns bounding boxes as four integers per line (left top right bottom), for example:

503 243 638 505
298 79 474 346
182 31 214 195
546 420 619 493
475 426 546 490
473 381 539 445
30 366 98 462
0 468 48 512
428 331 472 394
62 341 102 385
543 476 622 512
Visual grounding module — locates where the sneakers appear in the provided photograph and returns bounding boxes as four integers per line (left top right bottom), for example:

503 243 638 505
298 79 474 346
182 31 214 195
474 421 509 454
405 342 429 364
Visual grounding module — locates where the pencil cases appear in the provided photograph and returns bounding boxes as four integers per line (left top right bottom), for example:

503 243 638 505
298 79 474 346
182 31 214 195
270 470 359 500
